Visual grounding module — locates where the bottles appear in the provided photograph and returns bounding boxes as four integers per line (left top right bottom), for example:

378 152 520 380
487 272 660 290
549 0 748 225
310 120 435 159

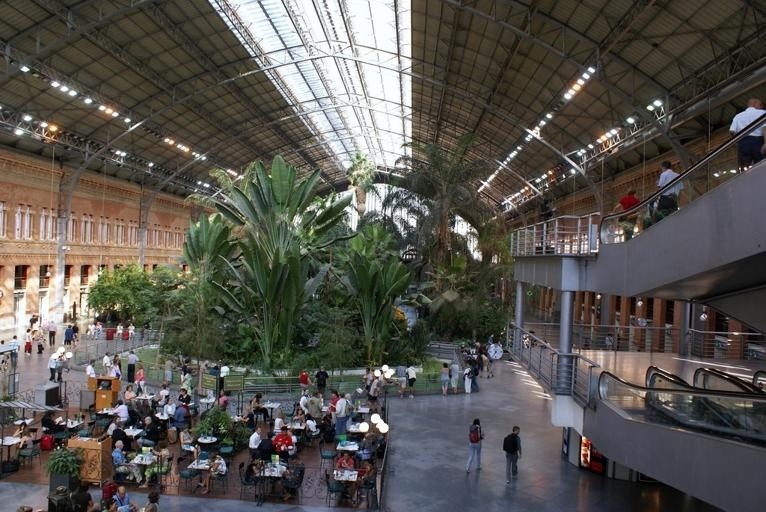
101 500 108 512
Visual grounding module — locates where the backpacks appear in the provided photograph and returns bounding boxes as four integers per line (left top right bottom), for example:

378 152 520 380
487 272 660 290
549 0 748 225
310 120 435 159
503 434 518 453
469 425 479 443
467 371 474 378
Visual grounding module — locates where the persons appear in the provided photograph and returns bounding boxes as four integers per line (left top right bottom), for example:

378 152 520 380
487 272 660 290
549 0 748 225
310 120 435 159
606 334 615 351
0 314 140 383
245 363 417 501
613 190 643 242
107 356 230 494
71 480 159 512
729 98 766 172
644 193 664 228
503 426 522 484
13 410 66 459
441 332 503 396
655 160 684 215
466 418 484 473
